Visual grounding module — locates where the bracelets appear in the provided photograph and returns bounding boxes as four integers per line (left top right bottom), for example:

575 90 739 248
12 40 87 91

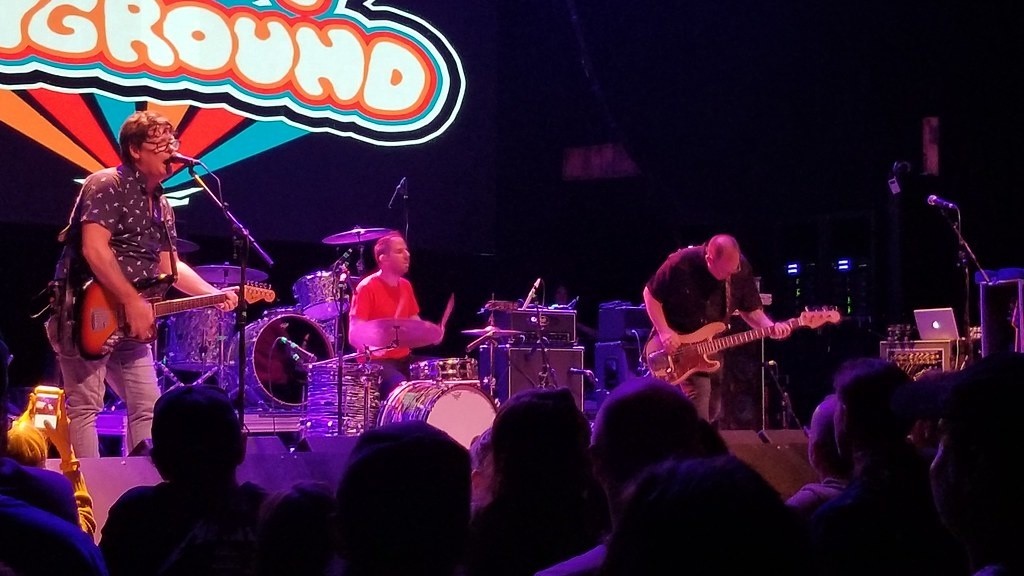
59 459 81 470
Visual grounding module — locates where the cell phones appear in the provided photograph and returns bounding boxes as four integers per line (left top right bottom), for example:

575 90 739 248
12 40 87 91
32 385 62 431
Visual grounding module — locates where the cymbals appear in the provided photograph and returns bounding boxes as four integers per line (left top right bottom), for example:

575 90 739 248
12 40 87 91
321 227 392 245
460 327 526 338
347 317 443 351
193 265 270 284
175 238 201 255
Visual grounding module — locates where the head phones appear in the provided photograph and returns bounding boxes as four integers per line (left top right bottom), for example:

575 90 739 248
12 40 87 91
149 383 249 481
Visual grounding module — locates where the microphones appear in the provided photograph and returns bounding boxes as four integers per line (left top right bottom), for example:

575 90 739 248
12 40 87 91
330 248 353 270
388 176 407 209
567 367 591 377
927 195 958 209
465 330 497 353
523 340 542 362
233 235 239 260
280 336 318 364
169 151 201 167
522 278 542 310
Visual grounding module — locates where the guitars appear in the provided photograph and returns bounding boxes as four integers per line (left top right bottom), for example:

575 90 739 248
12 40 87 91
643 304 842 387
71 272 276 361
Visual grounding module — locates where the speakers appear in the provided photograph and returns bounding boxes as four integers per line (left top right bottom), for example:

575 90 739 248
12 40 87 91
980 279 1024 357
478 345 584 412
126 436 285 457
594 340 650 412
290 436 360 453
718 429 764 446
598 300 654 341
758 429 813 444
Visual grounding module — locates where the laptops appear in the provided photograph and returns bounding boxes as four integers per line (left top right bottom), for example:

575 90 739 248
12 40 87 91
913 307 966 340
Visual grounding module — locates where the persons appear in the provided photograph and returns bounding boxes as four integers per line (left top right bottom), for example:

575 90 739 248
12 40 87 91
468 377 784 576
258 418 472 576
350 233 444 401
97 385 268 576
642 234 792 426
48 111 240 458
783 352 1024 576
0 340 108 576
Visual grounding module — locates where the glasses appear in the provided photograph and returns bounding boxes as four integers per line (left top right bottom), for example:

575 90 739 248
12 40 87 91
142 139 181 151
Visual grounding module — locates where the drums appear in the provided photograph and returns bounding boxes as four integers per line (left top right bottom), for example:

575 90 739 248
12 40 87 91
305 360 383 434
374 378 499 450
409 357 478 385
225 312 334 408
292 270 350 322
163 306 236 373
